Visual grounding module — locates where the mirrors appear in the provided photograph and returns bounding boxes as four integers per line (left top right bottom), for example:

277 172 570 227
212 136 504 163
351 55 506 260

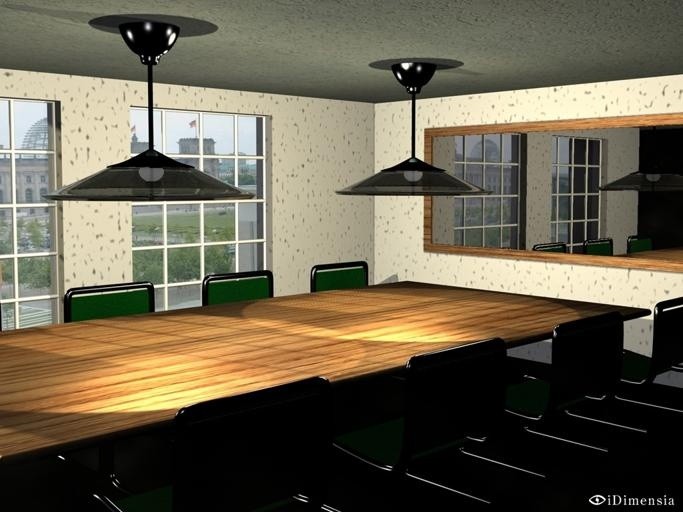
424 114 682 274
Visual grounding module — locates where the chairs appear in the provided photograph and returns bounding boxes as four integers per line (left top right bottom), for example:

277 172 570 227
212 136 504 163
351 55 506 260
616 297 683 415
536 241 565 251
45 372 333 511
581 239 615 254
510 310 647 453
309 259 367 292
200 269 274 306
60 283 156 323
335 337 545 510
624 235 653 251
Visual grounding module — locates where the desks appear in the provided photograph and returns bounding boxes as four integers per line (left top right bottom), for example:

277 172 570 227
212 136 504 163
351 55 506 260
2 279 618 460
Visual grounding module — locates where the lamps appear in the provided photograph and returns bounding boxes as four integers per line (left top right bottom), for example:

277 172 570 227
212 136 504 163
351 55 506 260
597 130 683 194
43 13 253 205
335 57 490 200
